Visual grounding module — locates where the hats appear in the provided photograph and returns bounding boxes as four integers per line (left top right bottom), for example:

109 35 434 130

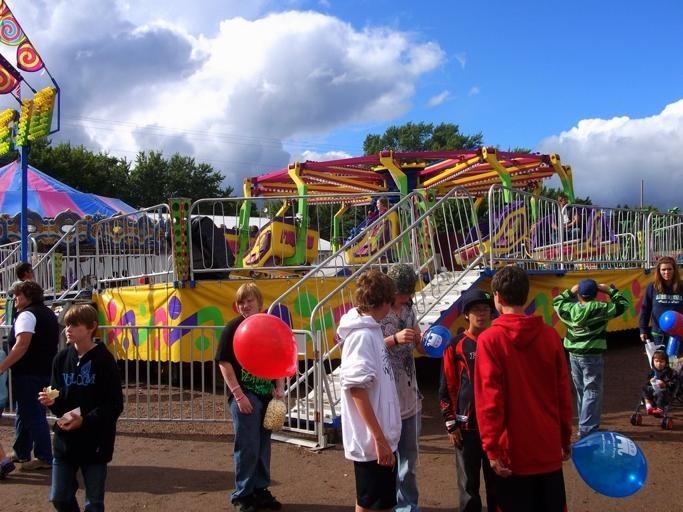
461 289 495 313
578 279 598 298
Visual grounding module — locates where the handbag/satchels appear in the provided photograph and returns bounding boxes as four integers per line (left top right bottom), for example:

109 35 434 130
263 391 287 432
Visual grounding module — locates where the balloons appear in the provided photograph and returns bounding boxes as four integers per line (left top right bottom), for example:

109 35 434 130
571 431 647 498
233 313 298 379
422 325 451 358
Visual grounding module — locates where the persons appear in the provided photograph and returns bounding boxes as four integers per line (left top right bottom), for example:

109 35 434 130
640 350 676 417
368 197 387 222
549 194 580 244
215 282 285 511
474 265 573 512
552 279 629 438
336 269 403 512
639 256 683 348
0 262 124 512
376 263 425 512
438 288 504 512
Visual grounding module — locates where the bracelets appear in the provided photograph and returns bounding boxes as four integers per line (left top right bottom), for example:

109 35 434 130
236 394 244 402
231 385 240 393
392 332 400 346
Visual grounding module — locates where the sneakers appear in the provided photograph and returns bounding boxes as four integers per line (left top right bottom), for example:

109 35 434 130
648 406 655 415
22 458 51 470
652 408 663 416
6 448 30 463
0 458 15 480
233 487 281 512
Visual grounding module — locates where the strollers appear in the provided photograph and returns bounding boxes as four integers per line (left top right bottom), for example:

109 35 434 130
628 366 680 436
